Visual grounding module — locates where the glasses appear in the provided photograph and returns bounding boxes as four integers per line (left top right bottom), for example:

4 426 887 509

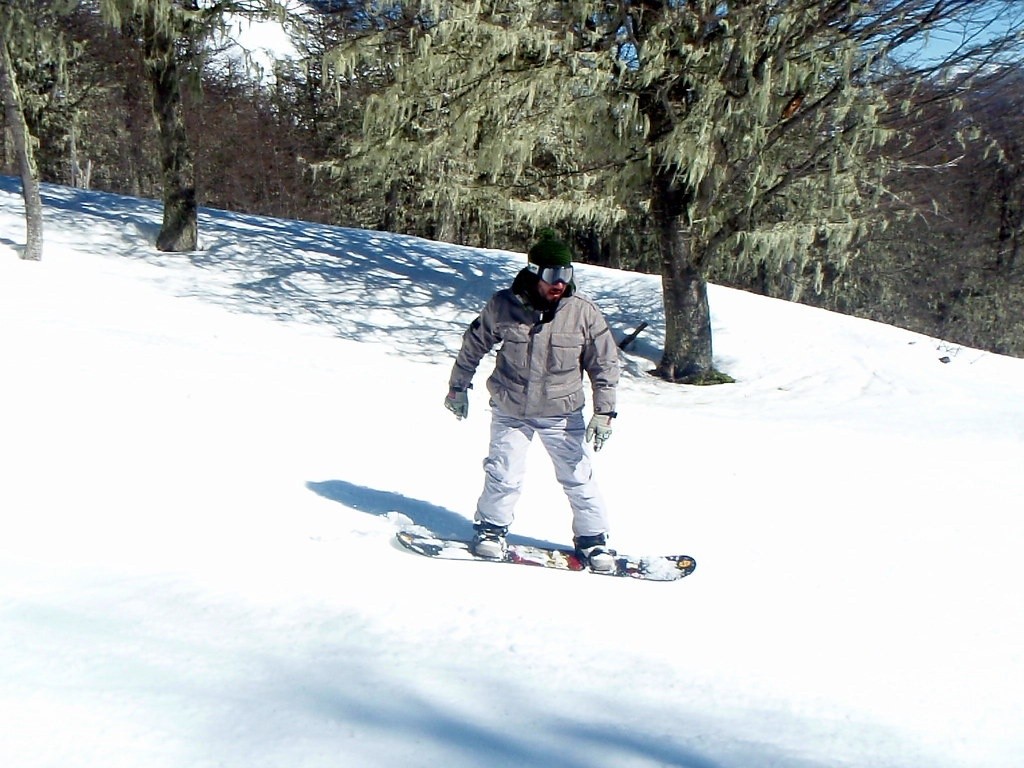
528 261 573 284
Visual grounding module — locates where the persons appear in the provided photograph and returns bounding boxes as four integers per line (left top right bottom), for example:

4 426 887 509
444 240 620 573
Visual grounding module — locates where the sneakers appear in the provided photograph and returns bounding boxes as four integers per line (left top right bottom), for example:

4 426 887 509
572 532 615 571
472 522 508 558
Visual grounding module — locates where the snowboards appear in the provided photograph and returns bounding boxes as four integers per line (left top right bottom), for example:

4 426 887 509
393 528 698 583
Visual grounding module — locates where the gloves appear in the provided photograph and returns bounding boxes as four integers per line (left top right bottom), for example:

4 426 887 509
444 386 468 421
586 412 612 452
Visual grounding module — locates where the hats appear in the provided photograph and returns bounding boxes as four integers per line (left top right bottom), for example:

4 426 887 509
528 229 571 268
